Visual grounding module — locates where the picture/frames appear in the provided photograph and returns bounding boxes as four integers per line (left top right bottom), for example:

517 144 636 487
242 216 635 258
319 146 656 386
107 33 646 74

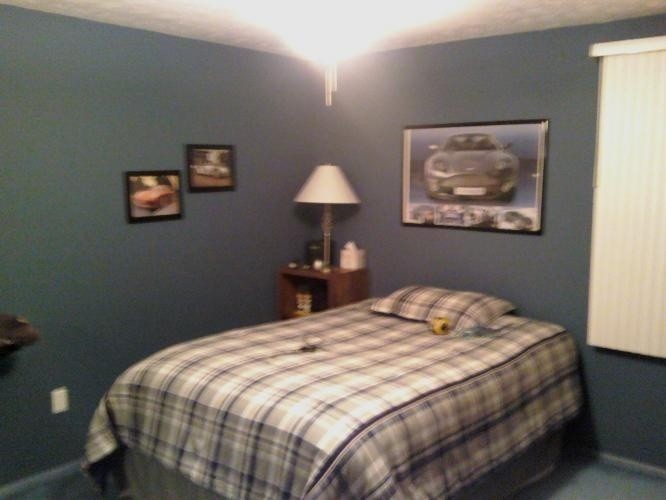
402 117 552 237
122 142 237 223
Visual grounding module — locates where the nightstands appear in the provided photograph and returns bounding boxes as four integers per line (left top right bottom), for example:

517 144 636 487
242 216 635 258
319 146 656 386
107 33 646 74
276 264 367 322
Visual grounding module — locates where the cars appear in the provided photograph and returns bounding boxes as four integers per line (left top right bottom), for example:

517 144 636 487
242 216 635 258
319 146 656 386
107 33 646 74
195 159 230 178
134 185 177 209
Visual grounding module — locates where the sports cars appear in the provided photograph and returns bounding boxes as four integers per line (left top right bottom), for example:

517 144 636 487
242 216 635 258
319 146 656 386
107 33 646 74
424 132 519 202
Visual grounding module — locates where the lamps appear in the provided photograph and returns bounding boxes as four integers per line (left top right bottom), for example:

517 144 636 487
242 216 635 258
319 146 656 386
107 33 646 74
294 164 360 270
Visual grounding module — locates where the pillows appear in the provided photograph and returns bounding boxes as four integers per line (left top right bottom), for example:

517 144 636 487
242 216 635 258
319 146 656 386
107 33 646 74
369 284 519 334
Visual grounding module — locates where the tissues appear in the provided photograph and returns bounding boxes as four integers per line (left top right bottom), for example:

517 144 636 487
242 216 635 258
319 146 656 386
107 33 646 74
340 240 366 270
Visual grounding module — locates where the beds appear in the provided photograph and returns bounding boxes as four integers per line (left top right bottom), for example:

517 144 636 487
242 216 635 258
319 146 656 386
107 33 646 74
112 297 580 499
